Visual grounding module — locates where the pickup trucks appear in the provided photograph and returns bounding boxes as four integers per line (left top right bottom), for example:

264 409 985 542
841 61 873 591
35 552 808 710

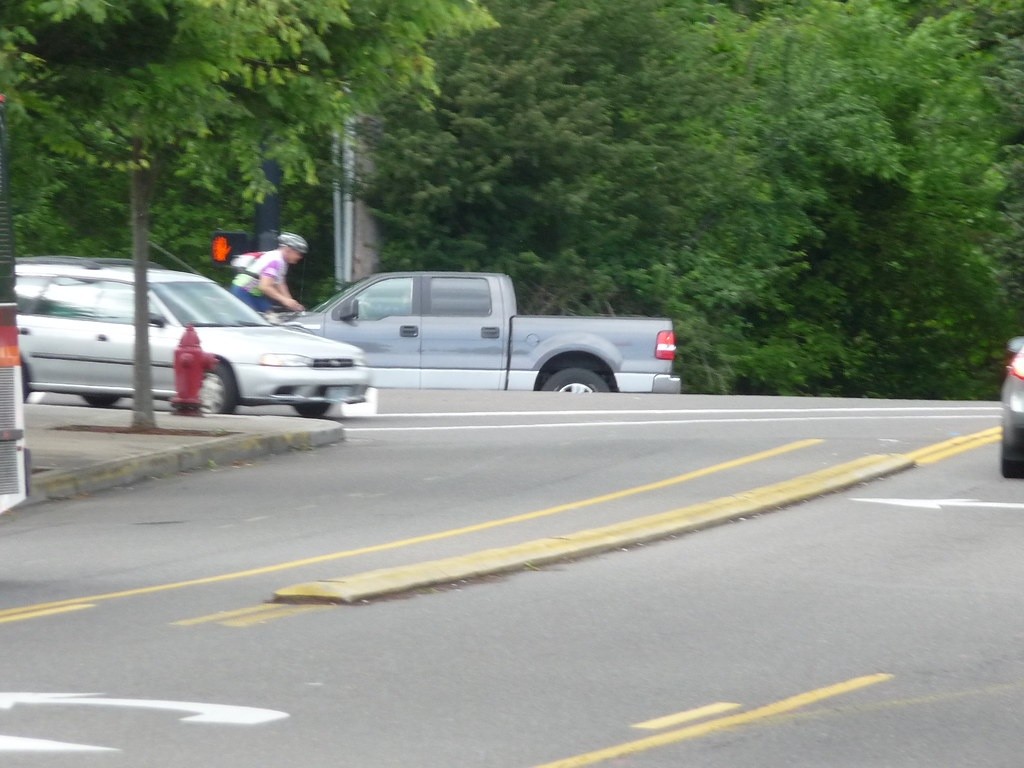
259 271 683 394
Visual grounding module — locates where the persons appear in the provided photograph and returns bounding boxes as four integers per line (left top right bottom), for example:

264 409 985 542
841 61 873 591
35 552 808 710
230 231 306 313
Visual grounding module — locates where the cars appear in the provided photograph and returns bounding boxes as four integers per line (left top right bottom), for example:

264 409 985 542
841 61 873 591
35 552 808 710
14 256 373 414
1000 337 1023 478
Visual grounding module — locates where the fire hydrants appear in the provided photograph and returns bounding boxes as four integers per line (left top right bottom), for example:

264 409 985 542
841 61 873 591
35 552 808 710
172 324 219 417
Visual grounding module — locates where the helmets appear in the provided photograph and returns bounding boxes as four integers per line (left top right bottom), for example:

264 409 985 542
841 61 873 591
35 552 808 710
277 231 309 256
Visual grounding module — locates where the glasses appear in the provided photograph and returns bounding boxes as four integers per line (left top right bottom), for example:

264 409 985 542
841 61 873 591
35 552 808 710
291 249 303 257
